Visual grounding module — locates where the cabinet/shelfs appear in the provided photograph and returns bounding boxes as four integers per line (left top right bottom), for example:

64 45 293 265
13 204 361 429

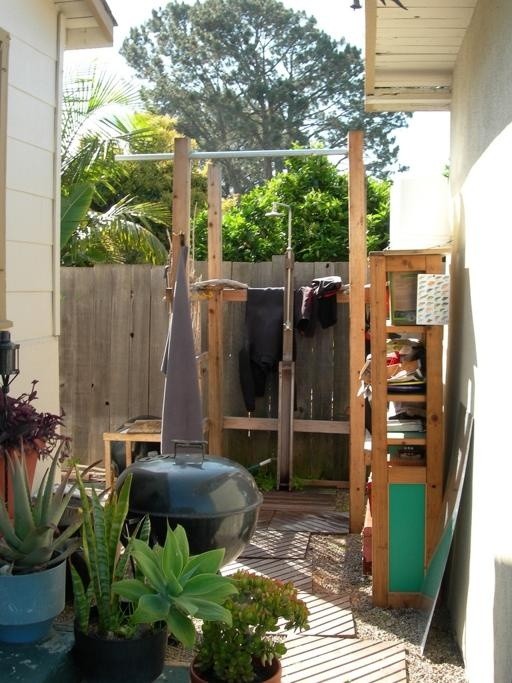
367 246 448 611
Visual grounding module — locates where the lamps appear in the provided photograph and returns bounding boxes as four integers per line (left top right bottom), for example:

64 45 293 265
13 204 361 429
0 320 20 393
